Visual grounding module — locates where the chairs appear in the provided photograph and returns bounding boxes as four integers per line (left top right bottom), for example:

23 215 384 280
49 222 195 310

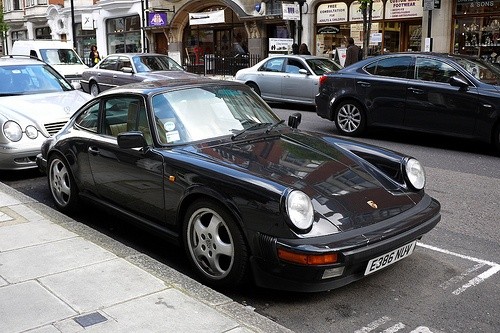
9 74 38 92
109 117 180 144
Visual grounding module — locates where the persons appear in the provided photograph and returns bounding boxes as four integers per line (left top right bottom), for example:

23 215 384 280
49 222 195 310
89 45 101 67
291 43 311 57
325 44 393 65
343 37 362 68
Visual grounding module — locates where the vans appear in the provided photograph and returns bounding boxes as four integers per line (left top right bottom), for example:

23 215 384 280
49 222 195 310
12 40 90 90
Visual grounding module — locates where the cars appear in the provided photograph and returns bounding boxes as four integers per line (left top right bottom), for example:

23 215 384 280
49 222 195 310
315 51 500 157
0 54 113 176
35 78 441 300
80 53 203 97
235 54 346 107
